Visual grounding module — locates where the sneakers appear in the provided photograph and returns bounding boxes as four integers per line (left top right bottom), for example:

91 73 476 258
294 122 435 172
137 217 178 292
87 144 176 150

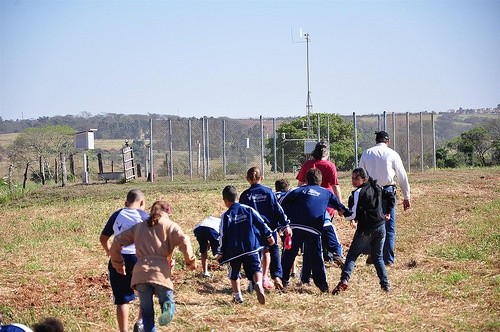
254 281 265 305
232 298 243 303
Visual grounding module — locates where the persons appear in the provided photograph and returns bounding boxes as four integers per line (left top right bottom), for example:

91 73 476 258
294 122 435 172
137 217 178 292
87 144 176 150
100 189 150 332
358 131 410 266
0 315 64 332
194 141 395 306
110 201 196 332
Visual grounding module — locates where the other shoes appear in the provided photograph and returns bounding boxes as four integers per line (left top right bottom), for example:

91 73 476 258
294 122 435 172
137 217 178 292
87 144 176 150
159 301 173 326
333 279 349 296
202 272 209 278
133 323 143 332
336 257 345 269
274 277 283 289
263 279 274 288
